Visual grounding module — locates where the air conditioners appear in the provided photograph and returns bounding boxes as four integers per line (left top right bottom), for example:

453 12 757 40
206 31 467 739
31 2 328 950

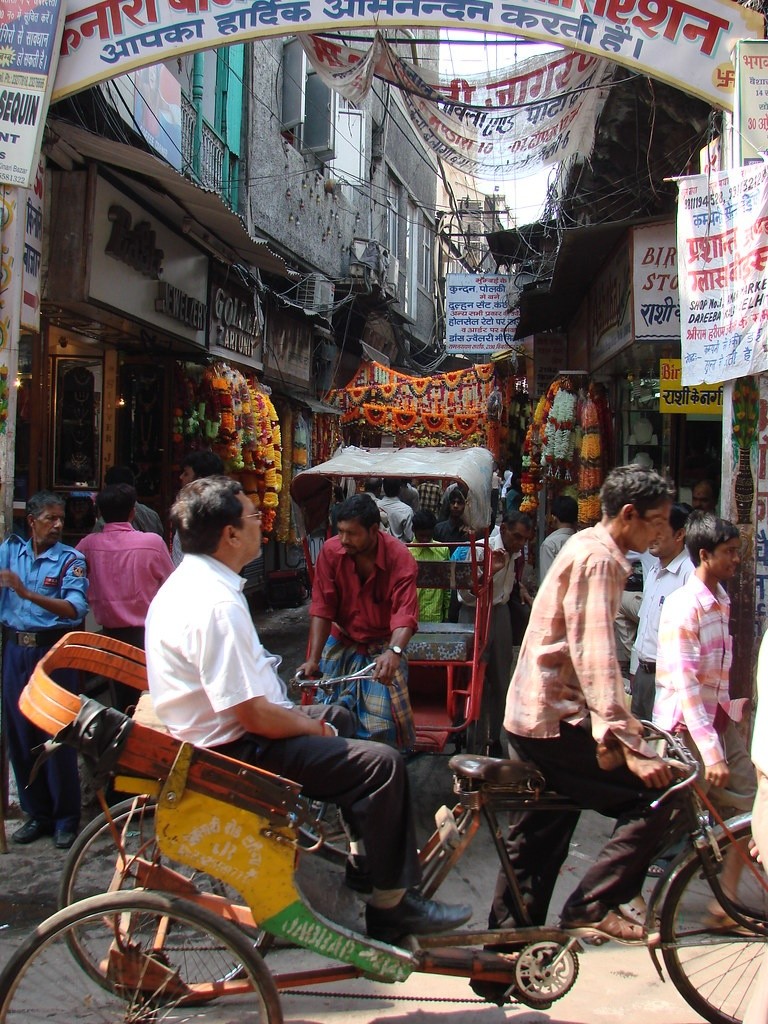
352 240 400 302
295 272 335 324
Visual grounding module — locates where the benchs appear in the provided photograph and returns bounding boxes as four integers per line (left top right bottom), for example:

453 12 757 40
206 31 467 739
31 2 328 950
388 562 481 665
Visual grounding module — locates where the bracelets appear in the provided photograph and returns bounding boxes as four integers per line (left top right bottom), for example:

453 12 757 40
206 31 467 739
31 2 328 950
317 718 326 737
324 722 338 736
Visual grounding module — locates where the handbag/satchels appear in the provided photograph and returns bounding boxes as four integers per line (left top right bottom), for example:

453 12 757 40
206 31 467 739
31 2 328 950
507 582 531 646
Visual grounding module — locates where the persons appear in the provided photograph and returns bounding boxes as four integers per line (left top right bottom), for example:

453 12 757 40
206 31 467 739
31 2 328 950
134 449 165 497
91 465 166 537
0 494 87 849
296 492 420 770
628 505 696 878
470 514 536 757
359 475 475 623
173 452 225 553
468 459 680 1008
144 476 475 939
538 495 580 583
461 509 538 759
71 481 174 823
747 625 768 903
491 467 514 516
651 510 758 938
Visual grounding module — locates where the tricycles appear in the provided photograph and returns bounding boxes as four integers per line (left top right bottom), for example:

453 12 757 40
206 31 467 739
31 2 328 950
1 629 768 1024
288 445 497 834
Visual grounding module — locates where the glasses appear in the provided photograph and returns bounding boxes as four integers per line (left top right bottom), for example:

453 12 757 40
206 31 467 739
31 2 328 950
239 507 264 520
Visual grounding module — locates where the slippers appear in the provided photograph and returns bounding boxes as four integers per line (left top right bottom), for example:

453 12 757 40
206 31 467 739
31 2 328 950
700 912 765 936
510 989 551 1011
618 895 661 933
560 910 660 946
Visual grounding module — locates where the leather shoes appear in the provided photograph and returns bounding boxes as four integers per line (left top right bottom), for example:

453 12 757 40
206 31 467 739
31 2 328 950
366 888 473 936
345 854 374 893
12 817 55 844
56 830 77 848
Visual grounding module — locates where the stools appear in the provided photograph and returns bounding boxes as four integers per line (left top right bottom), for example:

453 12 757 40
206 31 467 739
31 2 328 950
112 691 173 798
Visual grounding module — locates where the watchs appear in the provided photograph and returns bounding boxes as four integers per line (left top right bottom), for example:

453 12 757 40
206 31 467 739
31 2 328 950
387 645 404 657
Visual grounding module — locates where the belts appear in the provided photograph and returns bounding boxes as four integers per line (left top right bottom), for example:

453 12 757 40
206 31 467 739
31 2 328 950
638 659 656 673
4 622 84 647
